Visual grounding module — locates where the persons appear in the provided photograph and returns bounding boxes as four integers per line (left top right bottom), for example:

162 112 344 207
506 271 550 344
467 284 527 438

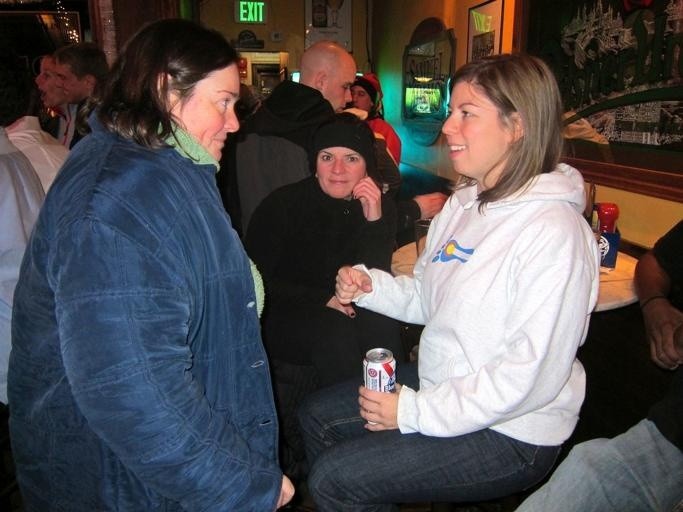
1 43 110 512
214 42 449 470
275 52 601 512
513 213 682 512
5 17 295 512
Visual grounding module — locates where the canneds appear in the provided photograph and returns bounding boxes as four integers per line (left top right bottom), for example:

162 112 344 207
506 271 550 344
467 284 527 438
362 348 397 426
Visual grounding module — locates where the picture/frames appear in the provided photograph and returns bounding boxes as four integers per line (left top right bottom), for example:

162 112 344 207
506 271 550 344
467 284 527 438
303 0 355 56
466 0 504 62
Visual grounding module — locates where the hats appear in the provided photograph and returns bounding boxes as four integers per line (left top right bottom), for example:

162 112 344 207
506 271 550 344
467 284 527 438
351 73 383 113
308 112 377 178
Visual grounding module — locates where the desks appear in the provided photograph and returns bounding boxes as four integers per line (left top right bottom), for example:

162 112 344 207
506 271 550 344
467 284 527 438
392 224 642 313
